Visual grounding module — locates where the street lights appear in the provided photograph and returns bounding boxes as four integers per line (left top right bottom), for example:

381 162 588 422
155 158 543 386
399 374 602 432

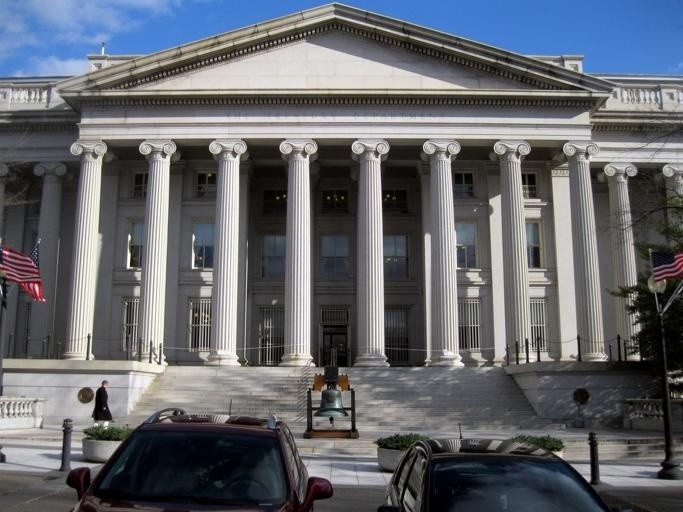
647 274 682 481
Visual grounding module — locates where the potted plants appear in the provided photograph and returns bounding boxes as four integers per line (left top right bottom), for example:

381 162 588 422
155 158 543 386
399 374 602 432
511 434 565 459
373 432 431 471
81 425 134 463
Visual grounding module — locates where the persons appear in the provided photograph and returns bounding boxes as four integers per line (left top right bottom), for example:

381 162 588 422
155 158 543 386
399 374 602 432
92 380 112 429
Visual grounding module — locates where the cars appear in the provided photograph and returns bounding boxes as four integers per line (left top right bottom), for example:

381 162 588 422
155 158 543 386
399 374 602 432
377 438 612 512
66 407 333 512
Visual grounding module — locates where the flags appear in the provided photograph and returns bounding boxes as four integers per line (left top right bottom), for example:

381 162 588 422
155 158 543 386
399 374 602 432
650 251 683 282
0 238 48 303
